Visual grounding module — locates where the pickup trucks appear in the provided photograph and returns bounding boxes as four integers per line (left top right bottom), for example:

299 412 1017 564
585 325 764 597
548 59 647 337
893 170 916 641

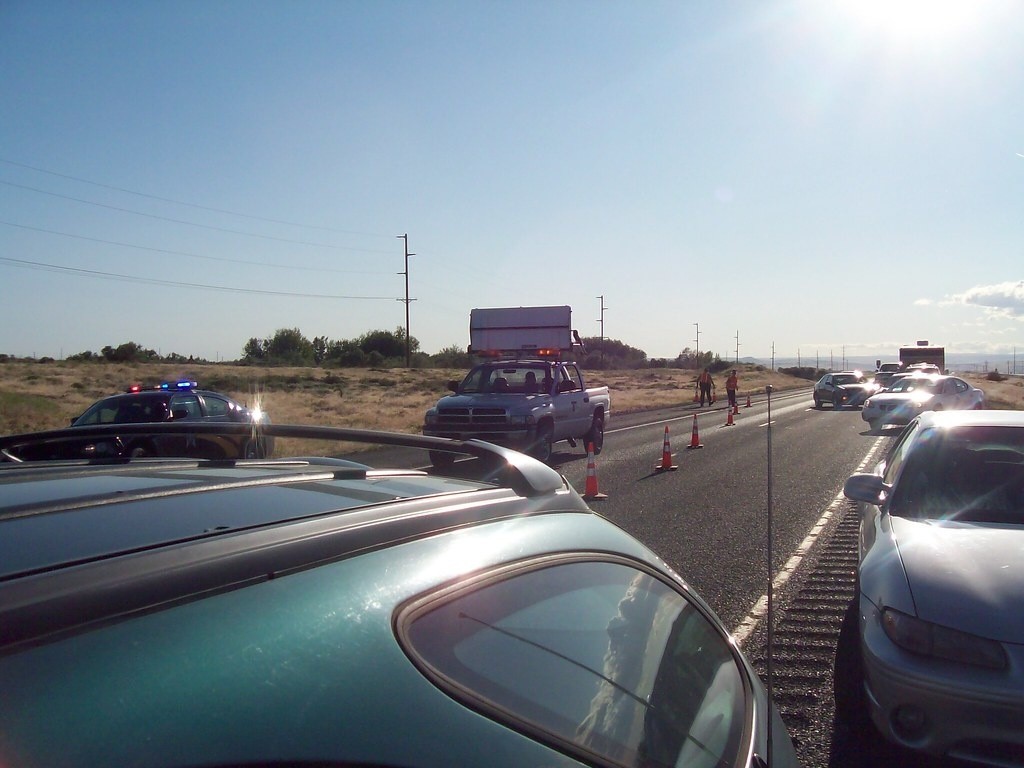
422 359 610 468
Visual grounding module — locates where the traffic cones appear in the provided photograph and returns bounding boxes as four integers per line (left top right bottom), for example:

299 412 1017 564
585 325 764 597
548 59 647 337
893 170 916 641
693 391 716 403
686 414 704 448
732 400 740 415
744 394 752 407
655 426 678 470
726 400 735 426
578 442 608 499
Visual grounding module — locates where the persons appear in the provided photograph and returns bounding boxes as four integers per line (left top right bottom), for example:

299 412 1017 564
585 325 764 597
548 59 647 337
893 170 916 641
525 372 537 391
696 368 715 406
726 370 738 406
917 449 993 509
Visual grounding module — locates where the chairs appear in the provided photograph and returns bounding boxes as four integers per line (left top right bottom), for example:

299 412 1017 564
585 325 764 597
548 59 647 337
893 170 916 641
938 446 984 500
490 378 509 392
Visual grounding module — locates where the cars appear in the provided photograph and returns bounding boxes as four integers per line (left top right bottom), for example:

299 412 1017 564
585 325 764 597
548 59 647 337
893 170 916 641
0 382 275 462
843 410 1024 768
0 422 803 768
813 372 878 410
876 361 905 387
862 375 984 430
